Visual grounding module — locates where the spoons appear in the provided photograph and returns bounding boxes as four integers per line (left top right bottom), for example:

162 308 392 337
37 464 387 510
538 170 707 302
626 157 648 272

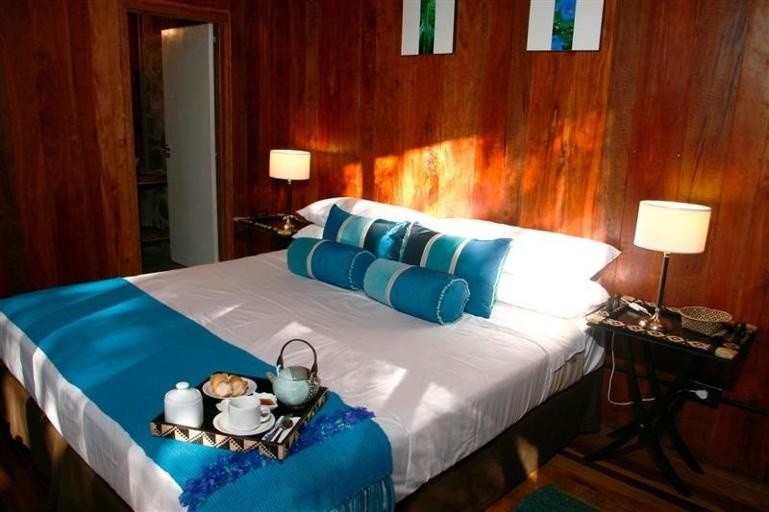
273 420 294 444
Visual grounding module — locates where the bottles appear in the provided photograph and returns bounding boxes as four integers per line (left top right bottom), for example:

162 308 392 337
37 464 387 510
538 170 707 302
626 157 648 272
162 380 206 429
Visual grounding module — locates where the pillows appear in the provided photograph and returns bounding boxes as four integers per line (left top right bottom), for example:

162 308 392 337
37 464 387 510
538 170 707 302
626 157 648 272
286 197 623 323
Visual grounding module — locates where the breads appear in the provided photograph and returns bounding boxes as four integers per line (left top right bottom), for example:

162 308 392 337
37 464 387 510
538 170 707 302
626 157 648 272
209 372 248 397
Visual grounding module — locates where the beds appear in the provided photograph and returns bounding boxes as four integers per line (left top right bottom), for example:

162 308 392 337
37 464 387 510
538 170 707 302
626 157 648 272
1 191 621 512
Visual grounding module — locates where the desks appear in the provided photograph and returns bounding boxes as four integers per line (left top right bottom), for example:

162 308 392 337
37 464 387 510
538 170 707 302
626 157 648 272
229 213 304 260
582 293 759 496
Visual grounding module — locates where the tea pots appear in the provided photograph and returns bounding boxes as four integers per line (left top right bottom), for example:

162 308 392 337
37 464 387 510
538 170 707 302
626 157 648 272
267 338 321 409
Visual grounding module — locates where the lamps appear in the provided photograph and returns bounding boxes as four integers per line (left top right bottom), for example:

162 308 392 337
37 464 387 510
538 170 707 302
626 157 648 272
628 197 717 330
267 147 313 234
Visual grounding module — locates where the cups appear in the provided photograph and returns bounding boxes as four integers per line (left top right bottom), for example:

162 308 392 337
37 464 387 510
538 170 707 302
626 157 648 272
227 397 270 431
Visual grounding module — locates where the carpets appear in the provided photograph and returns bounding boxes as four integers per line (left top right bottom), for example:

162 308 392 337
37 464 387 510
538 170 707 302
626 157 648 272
508 480 601 511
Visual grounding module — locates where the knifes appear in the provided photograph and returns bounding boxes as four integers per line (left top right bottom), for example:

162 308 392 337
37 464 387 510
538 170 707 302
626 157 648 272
263 412 296 442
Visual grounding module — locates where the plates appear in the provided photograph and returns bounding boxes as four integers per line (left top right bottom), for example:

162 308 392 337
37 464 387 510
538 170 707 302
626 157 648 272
252 393 279 411
202 377 259 399
213 411 276 437
